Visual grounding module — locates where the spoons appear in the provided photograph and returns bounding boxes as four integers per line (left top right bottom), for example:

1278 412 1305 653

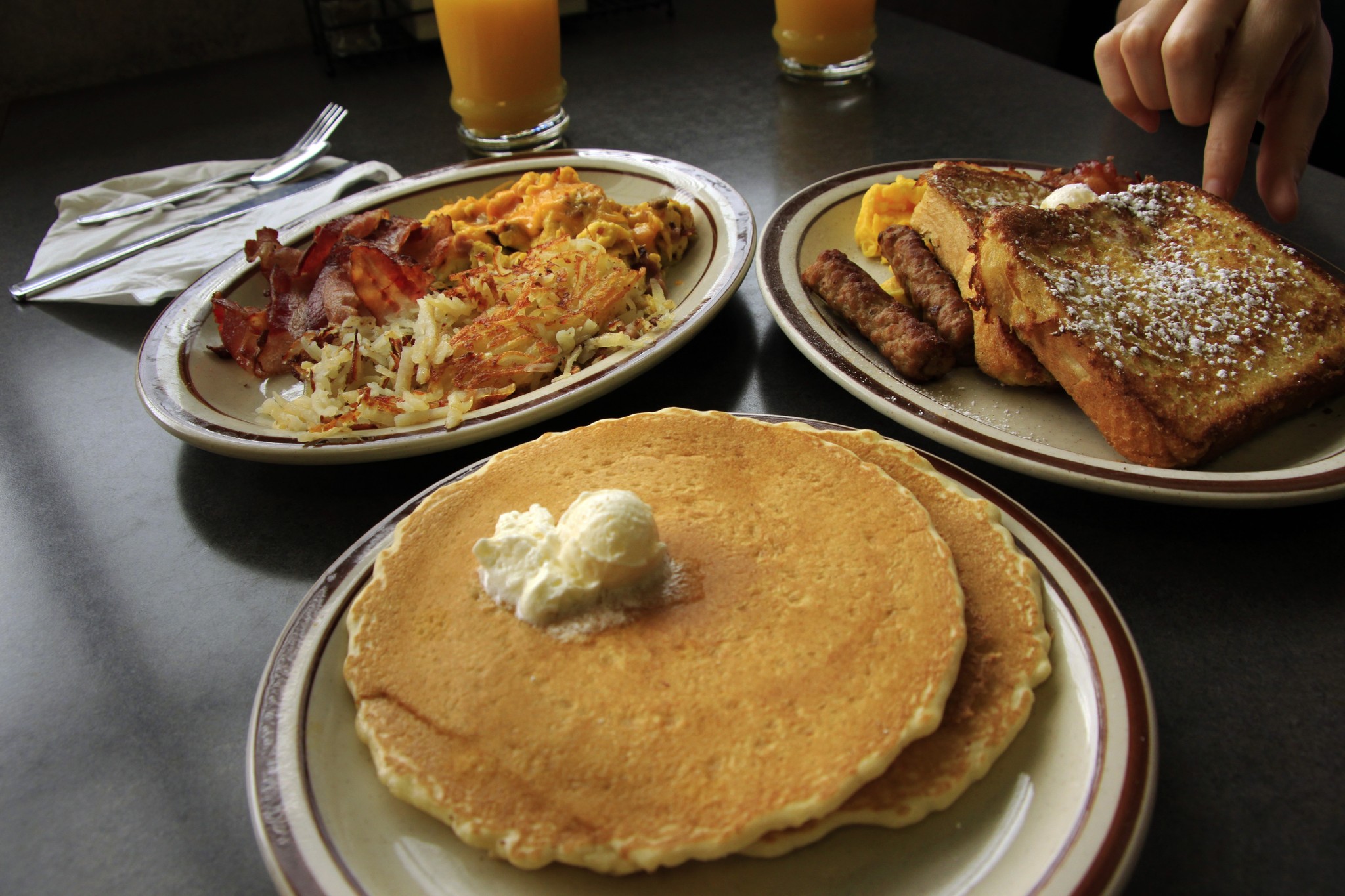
76 140 334 224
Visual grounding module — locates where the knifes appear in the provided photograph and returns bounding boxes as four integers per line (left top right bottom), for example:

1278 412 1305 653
8 160 359 301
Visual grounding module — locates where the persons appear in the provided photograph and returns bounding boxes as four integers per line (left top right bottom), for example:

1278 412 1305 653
1090 0 1340 216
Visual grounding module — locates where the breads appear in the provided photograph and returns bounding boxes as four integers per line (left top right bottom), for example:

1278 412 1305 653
341 409 1051 874
858 141 1345 473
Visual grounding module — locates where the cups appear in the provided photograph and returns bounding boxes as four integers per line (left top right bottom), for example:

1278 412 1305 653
433 0 571 158
773 0 878 88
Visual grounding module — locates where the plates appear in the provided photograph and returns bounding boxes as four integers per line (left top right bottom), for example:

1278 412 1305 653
755 158 1344 505
135 151 756 464
247 415 1160 896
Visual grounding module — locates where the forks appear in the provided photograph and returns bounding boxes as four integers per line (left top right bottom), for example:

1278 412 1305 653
168 102 350 194
